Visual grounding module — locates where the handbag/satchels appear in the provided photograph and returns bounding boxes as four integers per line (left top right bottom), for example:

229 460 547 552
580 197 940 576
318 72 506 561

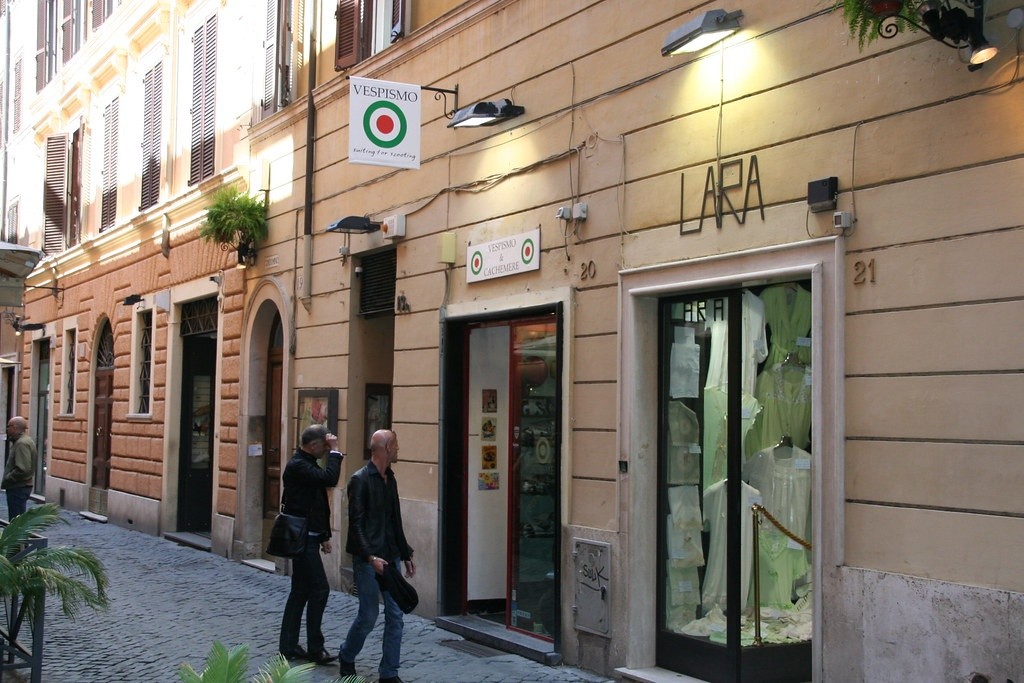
265 512 307 560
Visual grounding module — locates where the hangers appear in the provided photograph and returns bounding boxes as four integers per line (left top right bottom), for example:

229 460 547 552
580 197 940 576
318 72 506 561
785 282 797 292
774 424 794 450
781 340 806 369
717 381 745 396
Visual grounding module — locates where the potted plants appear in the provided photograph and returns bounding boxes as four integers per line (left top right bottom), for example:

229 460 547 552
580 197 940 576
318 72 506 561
198 180 270 246
812 0 944 54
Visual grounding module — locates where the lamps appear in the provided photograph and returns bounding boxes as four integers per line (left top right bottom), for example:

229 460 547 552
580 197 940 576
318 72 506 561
326 216 380 234
5 312 46 337
447 96 525 128
220 230 257 270
878 0 998 72
660 8 744 57
123 295 144 305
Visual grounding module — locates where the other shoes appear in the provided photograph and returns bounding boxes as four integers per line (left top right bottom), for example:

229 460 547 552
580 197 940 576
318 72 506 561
378 675 404 683
279 644 309 661
338 650 357 683
306 648 338 664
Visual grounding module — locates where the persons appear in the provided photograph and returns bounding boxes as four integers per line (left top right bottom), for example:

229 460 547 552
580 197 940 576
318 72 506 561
278 424 344 666
339 430 416 683
0 416 37 566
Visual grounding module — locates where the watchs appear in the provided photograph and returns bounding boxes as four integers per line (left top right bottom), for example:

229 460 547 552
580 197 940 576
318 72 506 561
370 556 378 564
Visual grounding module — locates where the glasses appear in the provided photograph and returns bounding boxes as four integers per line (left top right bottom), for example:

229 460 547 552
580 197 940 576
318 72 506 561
7 424 14 428
386 429 396 446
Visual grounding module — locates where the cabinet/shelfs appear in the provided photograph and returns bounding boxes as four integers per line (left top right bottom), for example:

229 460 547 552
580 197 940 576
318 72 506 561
518 329 556 585
